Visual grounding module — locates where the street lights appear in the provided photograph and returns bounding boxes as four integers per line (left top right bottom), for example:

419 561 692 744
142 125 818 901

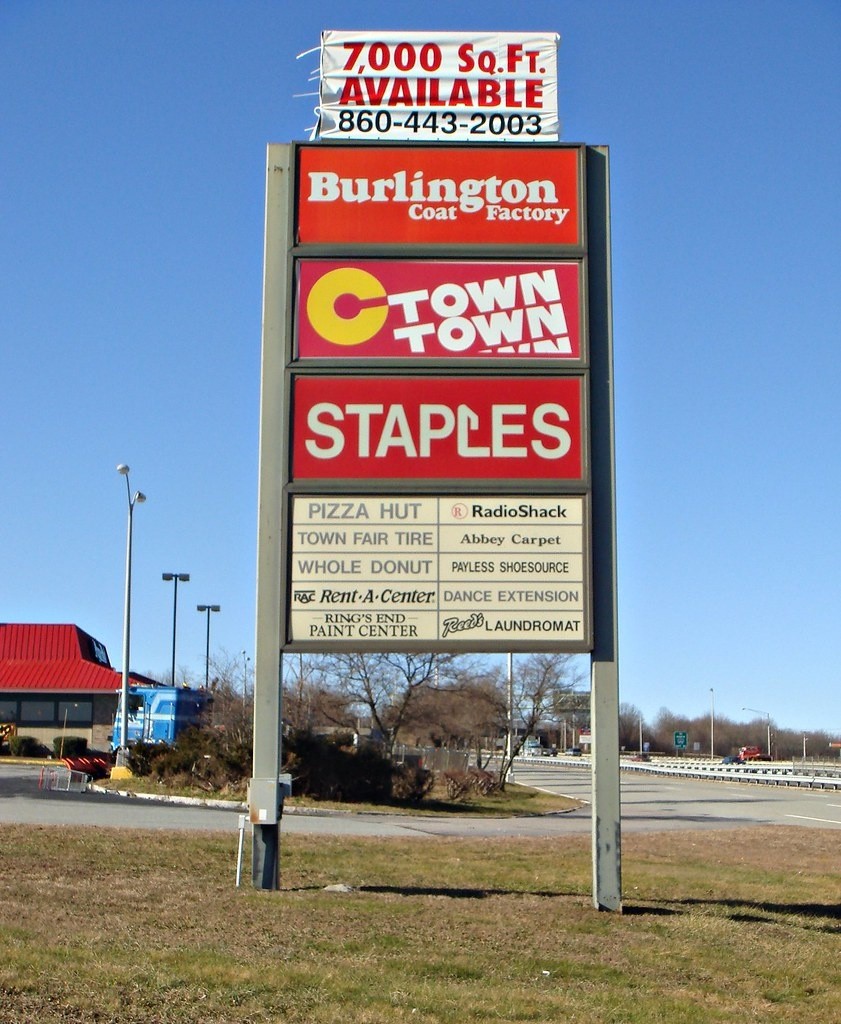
117 463 147 769
742 707 771 756
162 572 190 687
196 604 221 691
241 650 251 745
710 688 715 759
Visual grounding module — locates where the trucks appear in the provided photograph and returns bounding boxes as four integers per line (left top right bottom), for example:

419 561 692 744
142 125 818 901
738 745 774 761
106 681 215 766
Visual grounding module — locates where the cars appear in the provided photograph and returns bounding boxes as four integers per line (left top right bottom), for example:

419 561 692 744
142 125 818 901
721 755 746 765
565 748 581 756
632 754 652 762
543 748 558 757
